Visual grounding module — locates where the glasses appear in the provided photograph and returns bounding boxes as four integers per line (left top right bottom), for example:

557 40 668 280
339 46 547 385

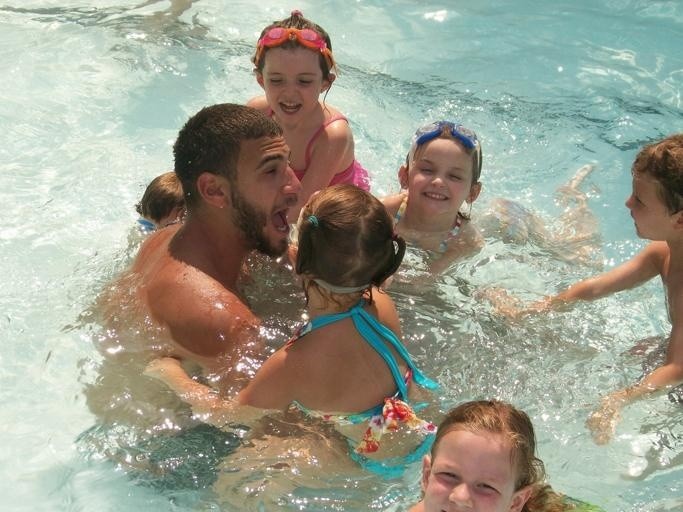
414 118 479 149
256 26 334 72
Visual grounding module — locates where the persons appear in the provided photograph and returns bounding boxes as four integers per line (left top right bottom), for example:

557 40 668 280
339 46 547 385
408 401 600 511
102 103 303 376
383 120 602 285
143 184 442 512
242 10 370 226
134 170 186 234
476 129 683 448
